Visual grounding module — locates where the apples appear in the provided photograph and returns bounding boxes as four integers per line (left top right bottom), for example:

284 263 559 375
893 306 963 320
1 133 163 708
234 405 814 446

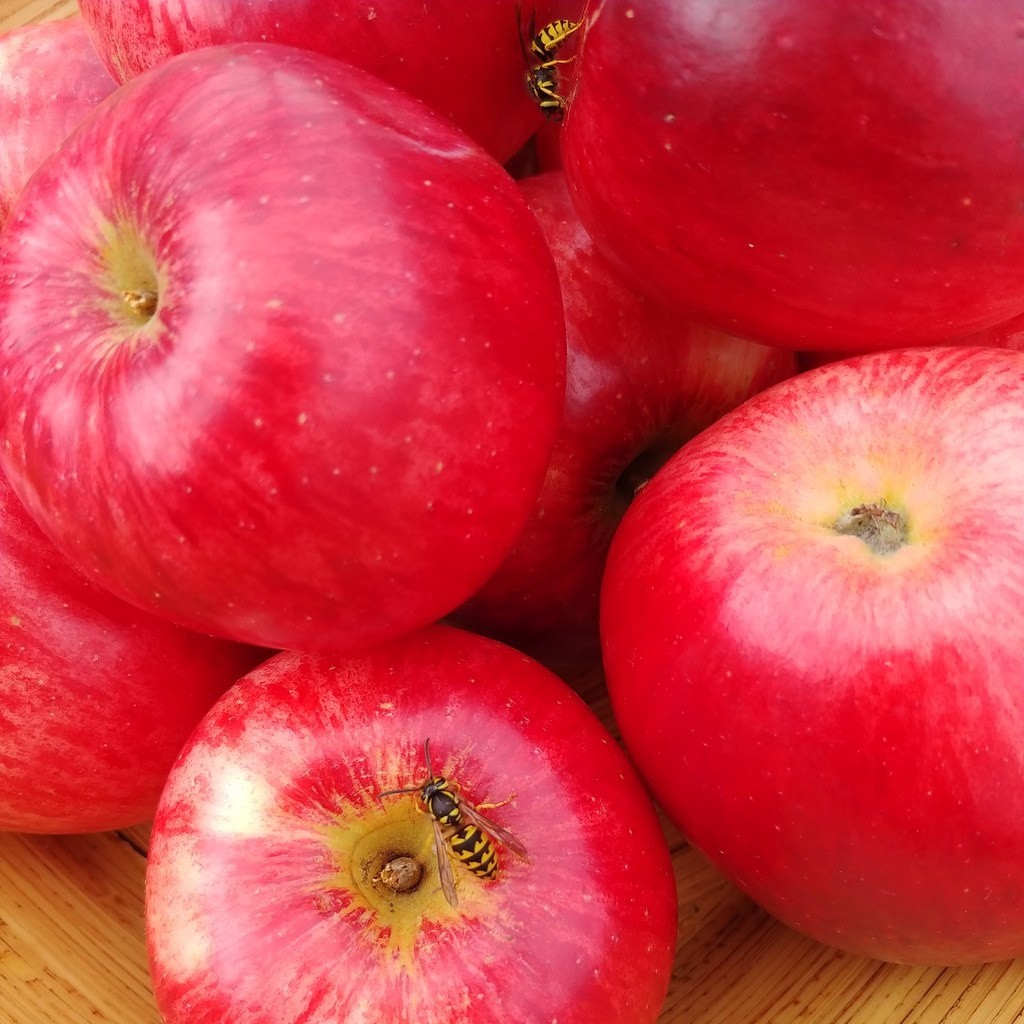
0 0 1023 1024
